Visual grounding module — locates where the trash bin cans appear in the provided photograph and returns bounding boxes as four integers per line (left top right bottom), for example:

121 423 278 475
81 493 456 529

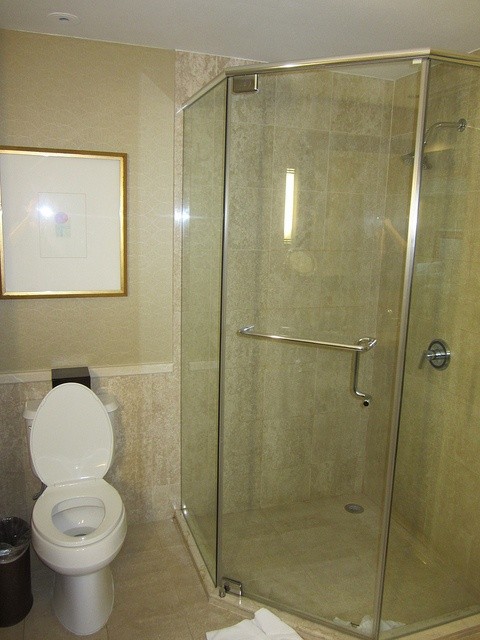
0 516 34 628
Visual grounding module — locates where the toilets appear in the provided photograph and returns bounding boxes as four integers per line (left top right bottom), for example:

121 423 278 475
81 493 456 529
29 382 129 637
